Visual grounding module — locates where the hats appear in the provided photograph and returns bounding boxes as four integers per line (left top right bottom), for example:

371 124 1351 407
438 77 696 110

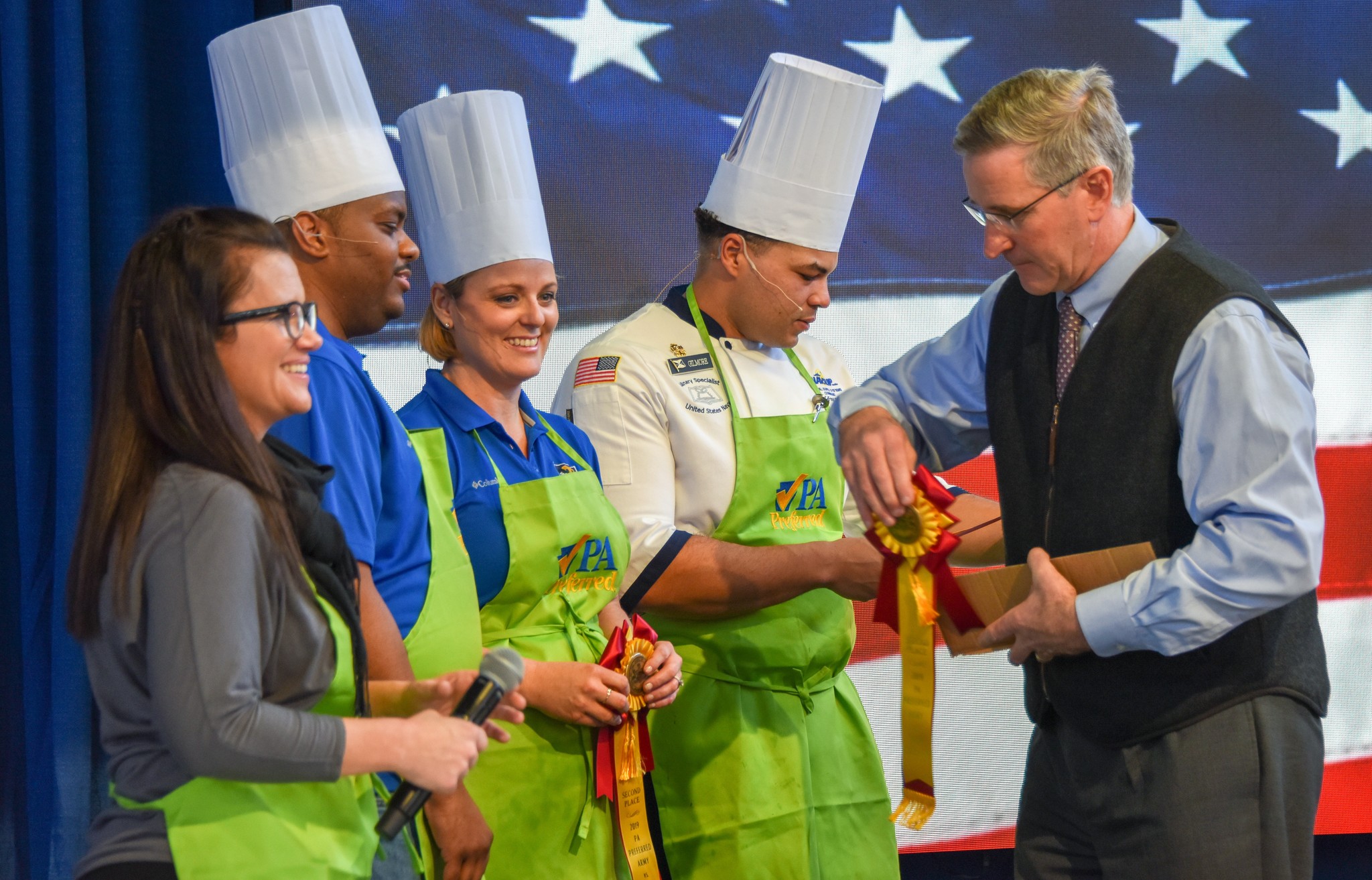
204 5 406 227
395 91 554 287
698 51 885 253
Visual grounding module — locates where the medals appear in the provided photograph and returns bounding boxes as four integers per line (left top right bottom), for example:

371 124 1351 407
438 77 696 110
614 636 659 712
869 487 940 558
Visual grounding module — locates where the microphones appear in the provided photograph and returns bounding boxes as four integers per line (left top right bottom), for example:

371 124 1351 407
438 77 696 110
372 644 524 839
745 248 804 310
453 306 510 351
316 231 377 243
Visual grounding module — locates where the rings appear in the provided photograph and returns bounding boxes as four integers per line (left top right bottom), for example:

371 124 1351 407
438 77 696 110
603 688 613 703
674 676 684 686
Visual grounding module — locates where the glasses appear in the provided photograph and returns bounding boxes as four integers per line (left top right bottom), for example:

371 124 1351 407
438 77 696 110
209 299 318 339
960 167 1094 235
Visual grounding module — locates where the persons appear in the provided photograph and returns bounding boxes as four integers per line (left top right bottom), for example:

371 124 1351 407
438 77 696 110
69 203 528 879
397 219 685 877
825 60 1329 879
549 152 903 879
241 129 503 880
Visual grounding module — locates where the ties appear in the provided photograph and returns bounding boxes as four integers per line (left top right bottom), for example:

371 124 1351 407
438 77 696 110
1057 295 1084 405
